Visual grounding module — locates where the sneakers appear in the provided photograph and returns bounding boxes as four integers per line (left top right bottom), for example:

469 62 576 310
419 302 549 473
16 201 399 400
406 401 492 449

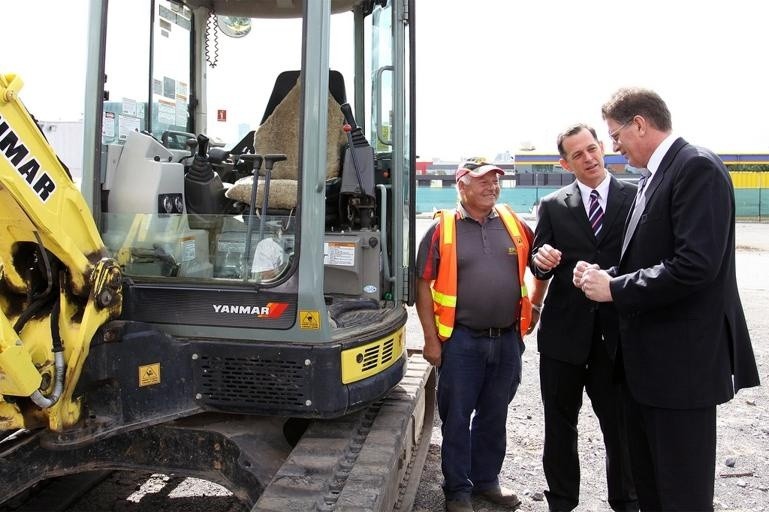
443 480 522 512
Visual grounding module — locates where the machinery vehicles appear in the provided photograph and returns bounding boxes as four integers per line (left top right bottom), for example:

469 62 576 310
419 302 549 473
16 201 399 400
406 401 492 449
0 0 440 511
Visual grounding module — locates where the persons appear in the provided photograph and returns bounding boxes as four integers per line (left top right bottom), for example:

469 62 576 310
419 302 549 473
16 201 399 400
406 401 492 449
530 122 640 512
414 155 549 512
572 86 762 512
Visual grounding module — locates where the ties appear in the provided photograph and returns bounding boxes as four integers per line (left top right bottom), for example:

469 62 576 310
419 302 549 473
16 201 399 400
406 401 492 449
619 168 652 268
588 189 605 240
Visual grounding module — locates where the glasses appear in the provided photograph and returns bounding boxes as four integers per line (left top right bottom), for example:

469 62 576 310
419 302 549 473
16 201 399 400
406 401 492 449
607 118 634 146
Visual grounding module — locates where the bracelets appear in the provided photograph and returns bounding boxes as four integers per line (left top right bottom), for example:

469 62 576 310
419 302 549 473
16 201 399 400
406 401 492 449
531 303 542 314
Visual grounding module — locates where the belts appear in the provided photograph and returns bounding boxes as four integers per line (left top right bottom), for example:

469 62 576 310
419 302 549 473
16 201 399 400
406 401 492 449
452 318 517 337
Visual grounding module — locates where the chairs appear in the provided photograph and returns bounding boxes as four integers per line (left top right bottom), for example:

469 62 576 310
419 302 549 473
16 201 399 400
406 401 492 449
223 68 349 232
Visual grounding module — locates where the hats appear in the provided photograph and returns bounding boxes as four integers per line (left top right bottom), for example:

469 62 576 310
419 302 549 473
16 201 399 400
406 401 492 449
453 155 506 184
